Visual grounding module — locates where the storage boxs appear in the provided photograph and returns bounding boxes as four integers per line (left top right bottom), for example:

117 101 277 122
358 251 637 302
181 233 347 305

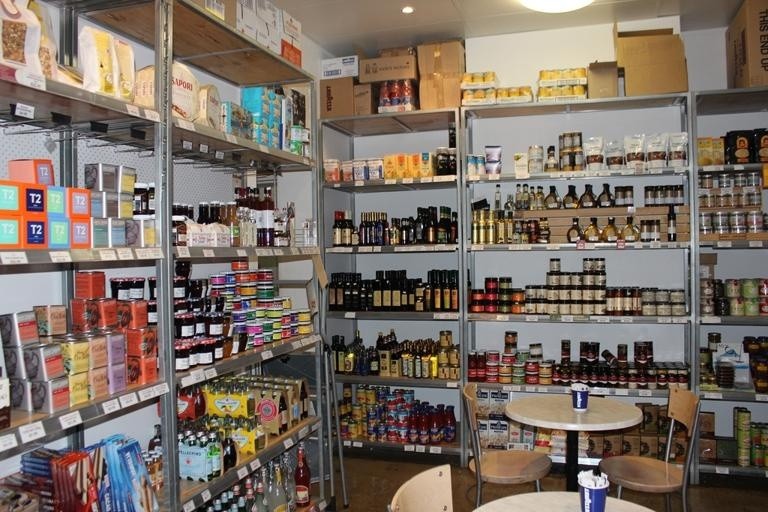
613 19 689 97
418 41 465 109
725 0 768 88
587 62 620 100
359 55 417 82
237 0 257 40
281 9 301 68
320 77 354 117
321 55 359 80
193 0 237 28
379 48 416 57
354 83 377 116
257 0 281 55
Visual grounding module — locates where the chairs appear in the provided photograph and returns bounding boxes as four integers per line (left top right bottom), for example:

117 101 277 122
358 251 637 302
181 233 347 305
388 464 453 512
599 385 701 512
463 383 553 506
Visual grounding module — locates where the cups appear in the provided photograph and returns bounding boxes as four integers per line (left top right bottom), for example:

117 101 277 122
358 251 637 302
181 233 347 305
576 471 609 512
570 383 590 412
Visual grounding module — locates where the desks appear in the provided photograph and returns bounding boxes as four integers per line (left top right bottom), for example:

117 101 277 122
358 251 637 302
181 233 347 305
470 492 654 512
506 395 643 490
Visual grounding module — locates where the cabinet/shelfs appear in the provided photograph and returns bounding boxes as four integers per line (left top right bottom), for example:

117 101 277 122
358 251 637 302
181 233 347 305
4 0 174 512
317 107 464 470
460 91 696 490
690 85 768 486
168 0 328 512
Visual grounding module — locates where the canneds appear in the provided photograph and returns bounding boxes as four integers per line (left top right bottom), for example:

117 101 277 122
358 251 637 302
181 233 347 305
331 381 419 444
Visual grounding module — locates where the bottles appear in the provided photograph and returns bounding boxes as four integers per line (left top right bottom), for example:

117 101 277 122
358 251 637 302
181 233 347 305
699 166 768 393
444 404 456 441
326 202 458 249
563 132 573 148
736 429 752 449
176 387 259 480
213 498 222 512
736 449 751 467
377 77 416 114
236 184 260 249
208 201 223 225
296 446 311 506
563 148 574 170
762 429 767 447
325 262 460 313
469 184 690 245
221 492 228 511
206 504 213 511
544 143 558 172
197 202 210 224
764 450 768 468
573 132 582 147
528 159 544 174
272 463 288 512
752 448 763 467
195 376 310 450
528 145 543 160
559 135 563 150
245 489 254 511
238 497 245 512
573 147 584 171
419 412 429 444
430 408 440 444
436 403 446 422
233 484 240 500
131 181 156 215
225 201 240 247
326 318 462 381
559 150 564 170
254 480 268 512
467 248 687 319
752 426 761 445
230 503 238 512
173 202 194 246
468 325 692 393
736 410 753 431
245 477 254 490
262 186 276 246
